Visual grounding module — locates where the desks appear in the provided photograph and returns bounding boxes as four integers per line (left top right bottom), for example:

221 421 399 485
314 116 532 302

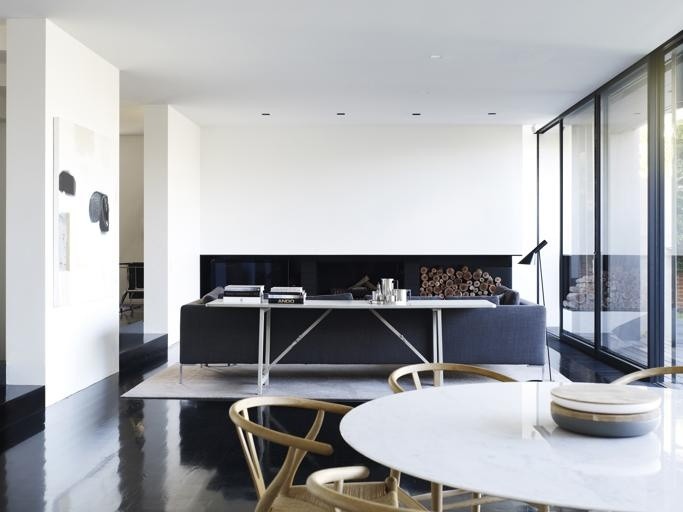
336 383 681 511
203 298 496 396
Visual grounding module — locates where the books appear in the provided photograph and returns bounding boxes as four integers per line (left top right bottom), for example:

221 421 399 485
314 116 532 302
266 298 306 303
269 290 305 296
222 295 264 303
224 291 263 297
267 292 306 298
270 286 303 292
223 285 265 292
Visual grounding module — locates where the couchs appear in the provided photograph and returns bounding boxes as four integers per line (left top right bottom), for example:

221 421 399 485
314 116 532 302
176 285 546 366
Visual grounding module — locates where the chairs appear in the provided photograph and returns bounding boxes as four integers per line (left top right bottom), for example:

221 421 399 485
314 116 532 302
228 397 403 512
305 466 421 511
388 362 522 511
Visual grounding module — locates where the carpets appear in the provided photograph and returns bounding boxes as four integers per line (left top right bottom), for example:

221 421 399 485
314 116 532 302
120 360 577 398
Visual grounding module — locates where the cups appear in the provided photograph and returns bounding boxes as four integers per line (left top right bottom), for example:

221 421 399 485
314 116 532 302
371 278 411 305
